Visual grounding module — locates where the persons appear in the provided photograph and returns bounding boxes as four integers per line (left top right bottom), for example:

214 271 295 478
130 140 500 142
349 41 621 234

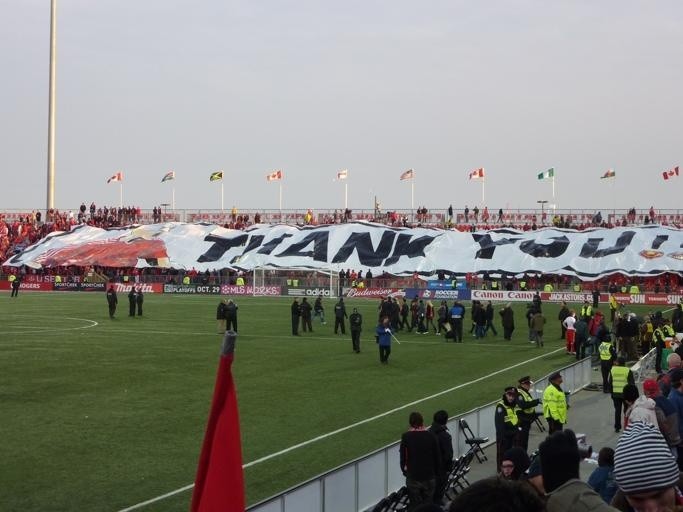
1 202 683 367
395 353 683 512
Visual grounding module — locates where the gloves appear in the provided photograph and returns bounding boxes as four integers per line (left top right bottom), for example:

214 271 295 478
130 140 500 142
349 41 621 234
539 429 579 494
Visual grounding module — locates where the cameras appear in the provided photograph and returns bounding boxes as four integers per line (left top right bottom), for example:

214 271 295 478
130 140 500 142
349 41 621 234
576 434 592 458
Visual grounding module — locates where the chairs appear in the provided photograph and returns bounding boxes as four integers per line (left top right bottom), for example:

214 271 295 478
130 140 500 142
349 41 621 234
457 416 491 465
360 448 477 511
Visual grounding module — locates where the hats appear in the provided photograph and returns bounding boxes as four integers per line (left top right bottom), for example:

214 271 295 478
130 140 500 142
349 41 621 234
500 447 530 480
505 377 532 394
549 371 560 381
615 396 680 493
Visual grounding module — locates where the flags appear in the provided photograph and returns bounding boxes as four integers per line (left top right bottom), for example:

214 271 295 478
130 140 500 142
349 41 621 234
468 168 482 179
188 333 247 512
106 172 122 185
336 169 348 181
209 170 222 182
662 166 678 180
599 169 614 179
399 169 415 180
160 172 173 184
265 170 281 182
537 167 553 180
